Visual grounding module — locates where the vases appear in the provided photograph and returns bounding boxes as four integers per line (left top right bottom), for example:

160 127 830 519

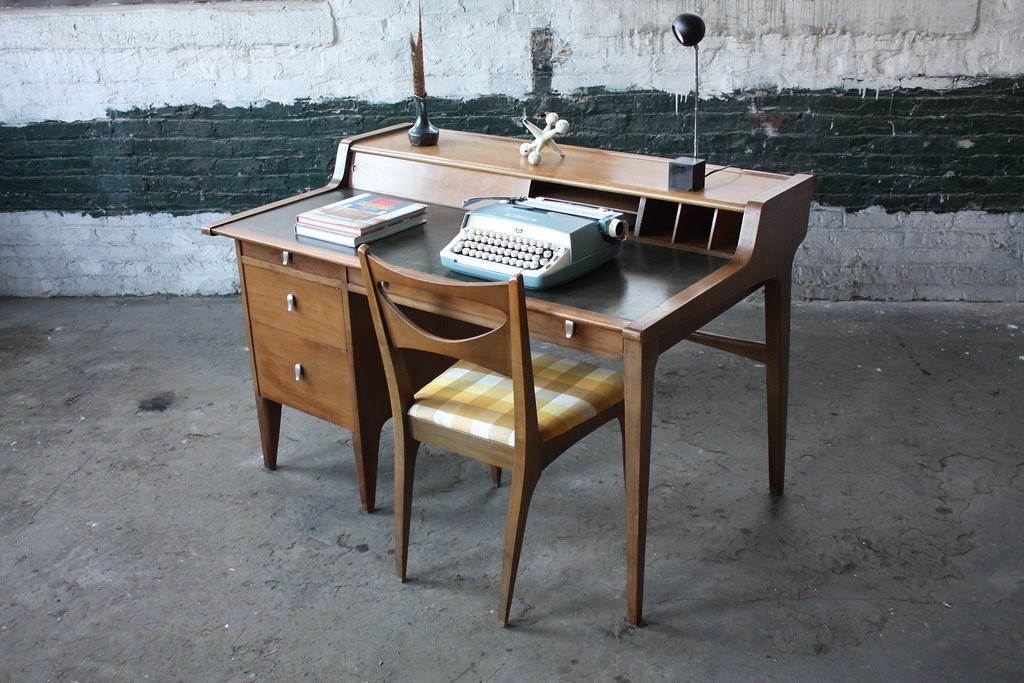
408 92 439 145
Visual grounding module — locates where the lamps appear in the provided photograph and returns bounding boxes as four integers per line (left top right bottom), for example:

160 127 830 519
667 14 705 192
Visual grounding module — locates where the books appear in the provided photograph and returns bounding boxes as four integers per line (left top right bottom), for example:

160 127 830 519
294 193 429 249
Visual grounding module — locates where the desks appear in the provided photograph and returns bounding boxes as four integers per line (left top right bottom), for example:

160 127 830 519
200 123 815 624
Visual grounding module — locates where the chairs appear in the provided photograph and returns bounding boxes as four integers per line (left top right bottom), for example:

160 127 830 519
356 242 626 629
